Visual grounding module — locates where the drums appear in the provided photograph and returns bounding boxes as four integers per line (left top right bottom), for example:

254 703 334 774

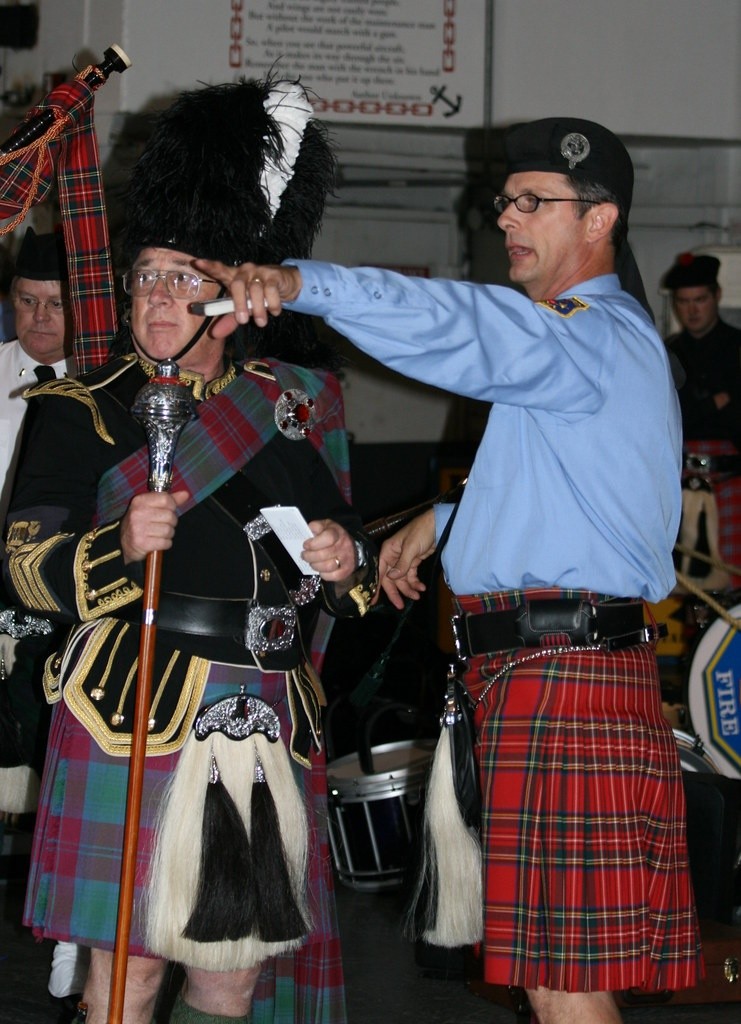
429 563 741 782
325 737 439 894
671 727 721 774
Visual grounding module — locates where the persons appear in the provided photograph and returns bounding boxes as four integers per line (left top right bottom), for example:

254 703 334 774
193 117 703 1024
1 84 382 1024
663 255 741 590
0 231 78 881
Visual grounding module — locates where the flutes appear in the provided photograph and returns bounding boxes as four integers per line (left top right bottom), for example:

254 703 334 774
0 40 134 224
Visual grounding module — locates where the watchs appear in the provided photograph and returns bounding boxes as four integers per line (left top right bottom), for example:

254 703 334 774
354 540 365 571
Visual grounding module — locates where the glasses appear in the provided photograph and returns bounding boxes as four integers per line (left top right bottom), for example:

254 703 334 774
492 192 601 214
14 296 63 314
122 269 222 300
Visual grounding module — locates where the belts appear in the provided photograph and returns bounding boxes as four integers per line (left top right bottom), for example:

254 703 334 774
681 452 741 475
99 589 319 655
455 598 668 660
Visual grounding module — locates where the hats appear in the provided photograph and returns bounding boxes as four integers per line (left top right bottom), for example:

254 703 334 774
502 116 634 219
663 253 720 289
15 226 70 281
116 78 333 266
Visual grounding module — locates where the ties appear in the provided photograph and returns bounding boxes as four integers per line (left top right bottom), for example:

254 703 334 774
34 365 57 385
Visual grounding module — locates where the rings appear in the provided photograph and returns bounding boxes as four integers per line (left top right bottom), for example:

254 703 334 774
249 278 263 289
335 557 340 568
